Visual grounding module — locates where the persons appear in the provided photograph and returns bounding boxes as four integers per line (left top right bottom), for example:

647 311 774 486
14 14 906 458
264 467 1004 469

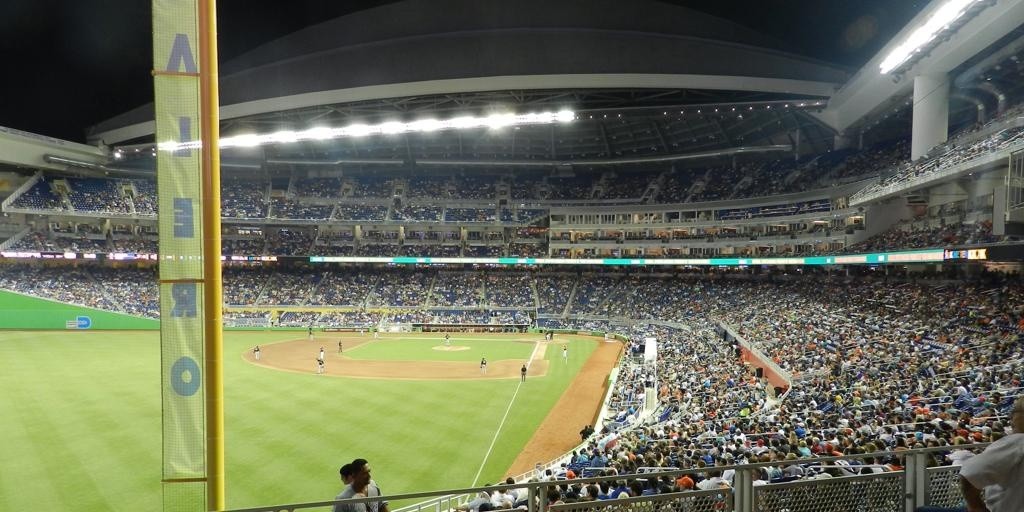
217 93 1023 337
339 463 352 484
1 172 161 321
460 337 1024 510
338 339 344 353
251 344 260 360
445 333 451 345
315 359 326 373
317 342 325 359
333 458 390 511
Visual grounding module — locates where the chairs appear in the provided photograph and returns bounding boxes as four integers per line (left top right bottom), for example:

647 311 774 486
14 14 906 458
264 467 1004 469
448 330 1024 512
0 109 1024 329
